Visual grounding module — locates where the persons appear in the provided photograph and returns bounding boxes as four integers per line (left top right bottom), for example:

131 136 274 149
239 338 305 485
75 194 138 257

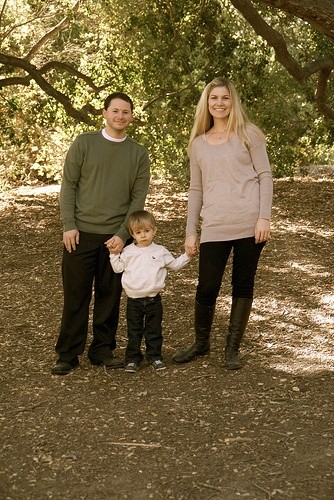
171 77 273 370
51 92 151 375
108 210 197 373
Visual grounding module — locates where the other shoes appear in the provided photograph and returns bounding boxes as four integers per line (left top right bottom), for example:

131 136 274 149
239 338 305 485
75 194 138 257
125 362 140 372
51 362 73 374
99 357 124 368
151 360 166 370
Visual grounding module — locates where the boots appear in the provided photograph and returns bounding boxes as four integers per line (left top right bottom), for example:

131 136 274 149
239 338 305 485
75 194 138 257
224 298 253 370
172 300 217 364
